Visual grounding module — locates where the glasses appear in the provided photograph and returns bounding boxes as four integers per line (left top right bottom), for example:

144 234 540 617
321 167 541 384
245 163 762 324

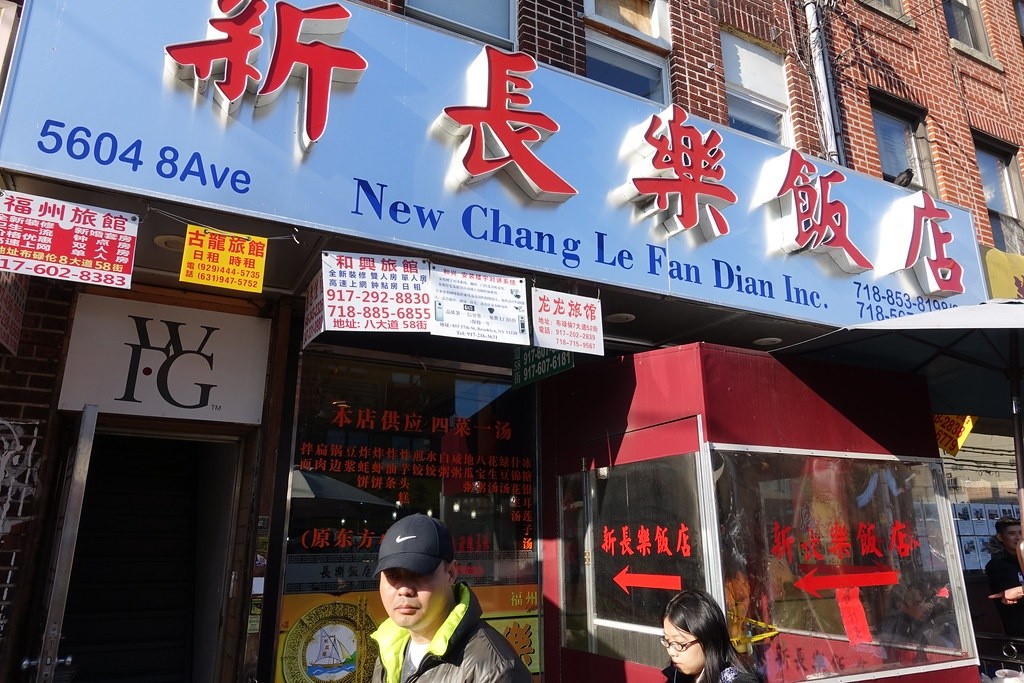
660 635 703 653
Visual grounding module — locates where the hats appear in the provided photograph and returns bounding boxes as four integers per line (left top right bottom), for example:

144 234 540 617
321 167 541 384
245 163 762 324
372 511 453 578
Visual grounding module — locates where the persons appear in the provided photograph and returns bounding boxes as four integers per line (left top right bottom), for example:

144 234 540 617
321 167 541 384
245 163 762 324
882 571 962 650
367 513 534 683
655 588 759 683
985 517 1024 666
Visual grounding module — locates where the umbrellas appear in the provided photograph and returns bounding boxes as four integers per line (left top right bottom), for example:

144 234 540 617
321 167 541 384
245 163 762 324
290 465 398 518
765 298 1024 490
